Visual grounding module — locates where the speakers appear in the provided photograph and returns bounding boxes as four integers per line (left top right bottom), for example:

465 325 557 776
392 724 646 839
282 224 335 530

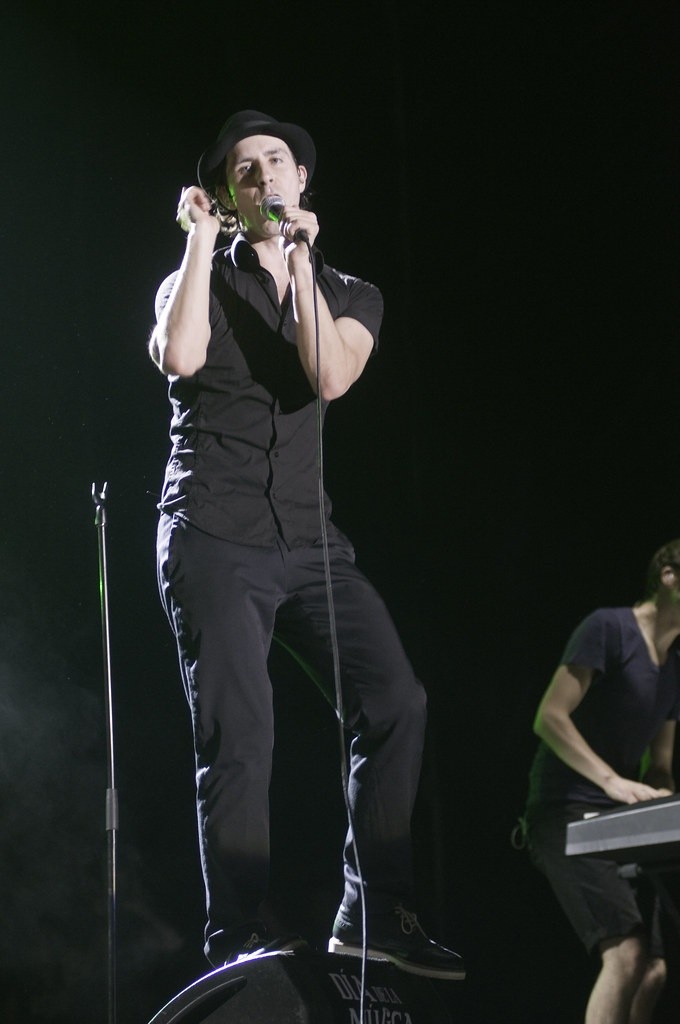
147 948 452 1024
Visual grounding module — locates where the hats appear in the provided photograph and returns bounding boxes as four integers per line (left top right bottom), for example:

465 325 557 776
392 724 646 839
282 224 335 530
198 109 316 215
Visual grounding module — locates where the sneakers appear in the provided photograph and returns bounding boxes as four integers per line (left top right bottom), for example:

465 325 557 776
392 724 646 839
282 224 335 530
327 904 465 979
221 930 323 965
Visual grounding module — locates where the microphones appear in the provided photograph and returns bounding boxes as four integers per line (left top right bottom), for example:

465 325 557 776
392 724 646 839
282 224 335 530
259 194 311 243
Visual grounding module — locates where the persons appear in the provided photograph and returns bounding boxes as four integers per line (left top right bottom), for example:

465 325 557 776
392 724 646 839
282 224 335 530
523 537 680 1024
148 110 468 980
